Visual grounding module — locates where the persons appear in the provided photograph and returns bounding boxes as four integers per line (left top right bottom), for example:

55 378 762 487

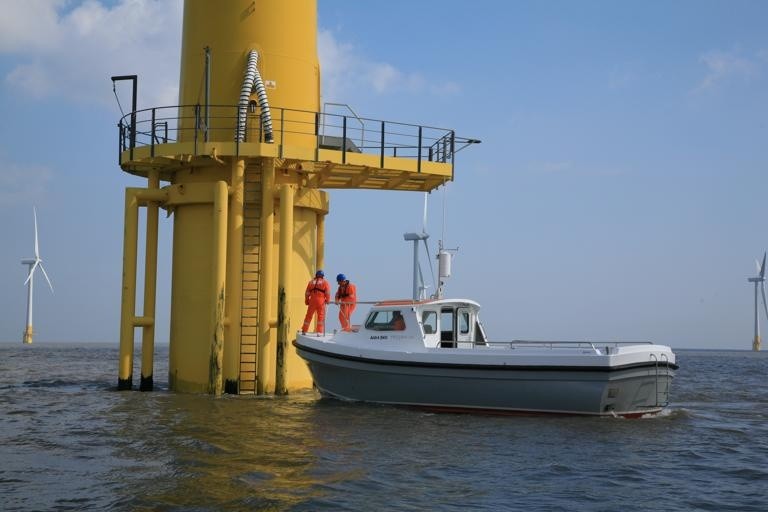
300 269 330 338
390 312 403 330
332 273 355 331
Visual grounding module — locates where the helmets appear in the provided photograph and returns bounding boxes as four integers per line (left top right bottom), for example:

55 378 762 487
315 271 323 276
336 274 345 281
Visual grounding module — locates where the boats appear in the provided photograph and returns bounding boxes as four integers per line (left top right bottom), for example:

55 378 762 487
292 188 681 422
